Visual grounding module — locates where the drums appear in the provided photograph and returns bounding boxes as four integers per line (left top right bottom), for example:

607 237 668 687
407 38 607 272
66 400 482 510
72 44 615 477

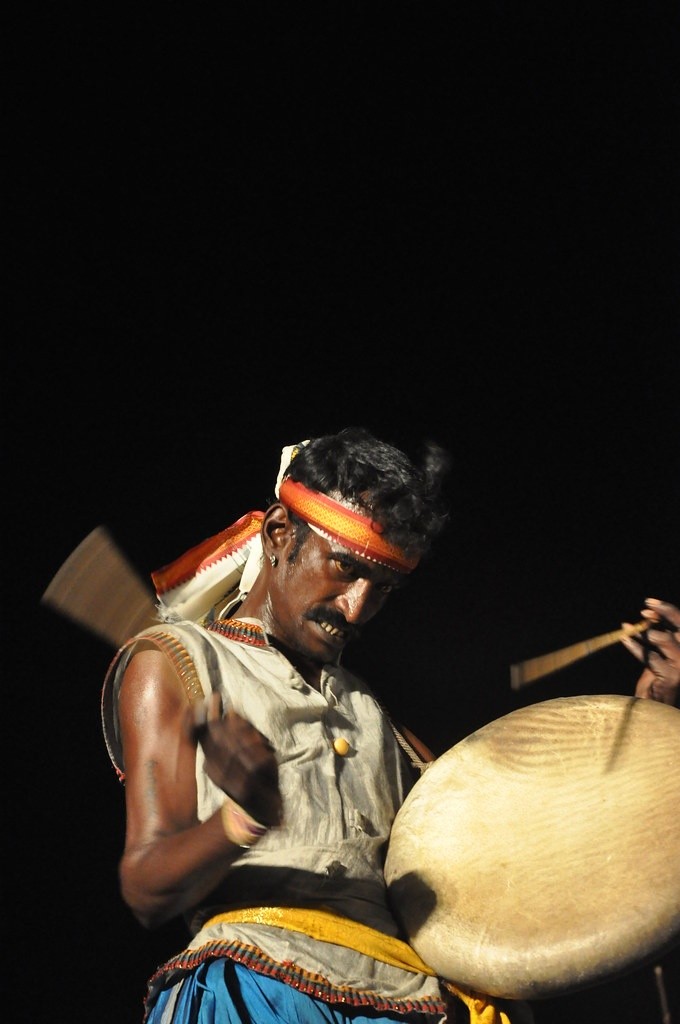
383 694 680 998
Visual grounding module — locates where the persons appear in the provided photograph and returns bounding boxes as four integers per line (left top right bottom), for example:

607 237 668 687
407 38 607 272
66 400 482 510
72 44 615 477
100 423 680 1024
